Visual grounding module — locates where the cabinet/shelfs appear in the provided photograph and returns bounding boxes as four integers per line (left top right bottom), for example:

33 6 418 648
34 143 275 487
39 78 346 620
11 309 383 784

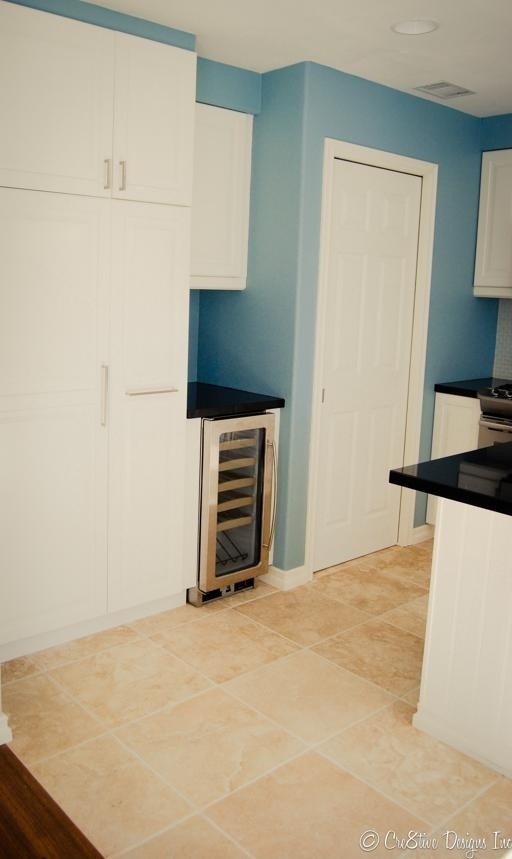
0 0 198 208
1 186 195 665
471 147 512 300
187 101 254 293
424 390 483 529
